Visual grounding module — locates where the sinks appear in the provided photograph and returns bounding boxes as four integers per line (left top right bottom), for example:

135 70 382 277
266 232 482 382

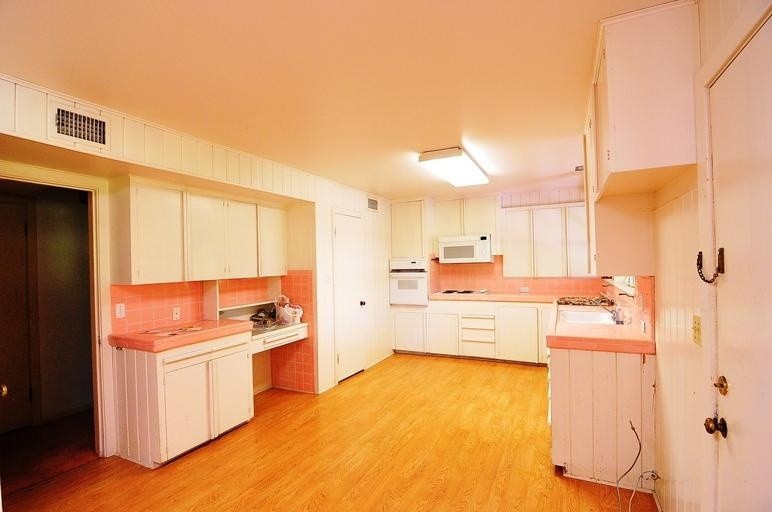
559 311 614 324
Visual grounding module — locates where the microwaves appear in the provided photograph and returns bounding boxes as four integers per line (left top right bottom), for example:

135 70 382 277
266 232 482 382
438 233 495 264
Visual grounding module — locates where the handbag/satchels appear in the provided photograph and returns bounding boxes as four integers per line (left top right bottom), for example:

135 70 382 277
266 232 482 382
275 295 303 326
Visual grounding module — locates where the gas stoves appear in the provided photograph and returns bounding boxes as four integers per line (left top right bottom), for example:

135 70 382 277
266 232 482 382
433 288 490 295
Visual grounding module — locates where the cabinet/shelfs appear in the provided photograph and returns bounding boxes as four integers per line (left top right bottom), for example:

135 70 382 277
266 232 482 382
501 202 590 278
107 172 186 286
591 1 699 201
434 192 497 259
392 300 460 357
251 326 309 355
391 199 423 259
257 200 289 277
460 301 495 359
495 301 555 364
186 185 257 283
113 331 255 470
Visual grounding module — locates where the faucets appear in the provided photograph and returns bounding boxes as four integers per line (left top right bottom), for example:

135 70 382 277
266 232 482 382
588 298 615 317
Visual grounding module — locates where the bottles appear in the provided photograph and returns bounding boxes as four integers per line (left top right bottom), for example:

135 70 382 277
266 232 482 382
615 301 624 325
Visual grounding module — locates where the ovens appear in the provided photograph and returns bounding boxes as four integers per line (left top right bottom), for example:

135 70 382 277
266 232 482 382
389 259 428 306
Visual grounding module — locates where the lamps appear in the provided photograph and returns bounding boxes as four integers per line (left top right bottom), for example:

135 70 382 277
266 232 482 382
418 147 490 189
